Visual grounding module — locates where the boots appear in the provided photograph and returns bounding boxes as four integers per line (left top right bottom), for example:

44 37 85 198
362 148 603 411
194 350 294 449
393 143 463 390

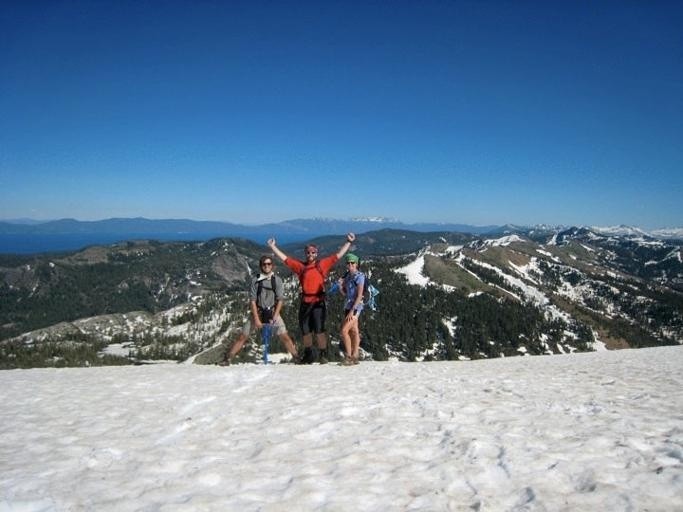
294 347 328 364
220 352 233 366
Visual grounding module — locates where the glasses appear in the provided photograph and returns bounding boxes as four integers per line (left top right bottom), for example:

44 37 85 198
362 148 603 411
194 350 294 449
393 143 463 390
306 252 315 254
347 262 355 264
264 263 271 266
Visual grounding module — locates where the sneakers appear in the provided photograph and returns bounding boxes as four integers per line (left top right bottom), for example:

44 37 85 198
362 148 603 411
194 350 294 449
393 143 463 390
337 355 359 365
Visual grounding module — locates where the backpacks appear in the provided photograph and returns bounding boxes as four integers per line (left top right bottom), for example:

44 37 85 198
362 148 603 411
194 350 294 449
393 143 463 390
354 273 379 312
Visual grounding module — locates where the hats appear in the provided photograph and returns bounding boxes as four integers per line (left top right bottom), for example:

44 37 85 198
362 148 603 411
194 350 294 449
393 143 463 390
346 254 358 264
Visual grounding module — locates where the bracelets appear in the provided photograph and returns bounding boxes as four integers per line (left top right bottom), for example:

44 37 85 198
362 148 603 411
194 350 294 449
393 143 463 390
346 240 352 243
350 306 356 310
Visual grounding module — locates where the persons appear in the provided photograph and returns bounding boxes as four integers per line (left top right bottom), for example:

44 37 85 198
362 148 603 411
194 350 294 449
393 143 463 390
265 232 356 364
337 254 368 367
220 255 300 366
